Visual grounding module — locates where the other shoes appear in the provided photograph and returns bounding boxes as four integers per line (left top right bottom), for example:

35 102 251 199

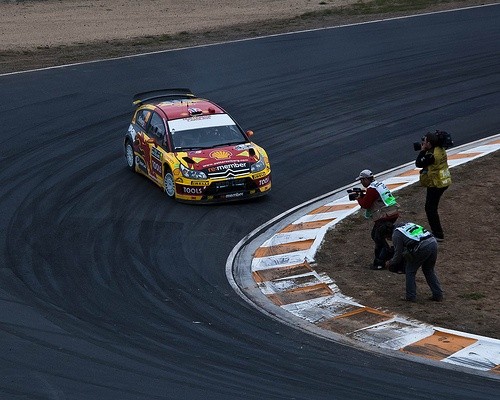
372 265 386 270
399 295 414 301
429 295 443 302
435 237 444 241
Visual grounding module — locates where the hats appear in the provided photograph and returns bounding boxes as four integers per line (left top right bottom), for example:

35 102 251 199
355 169 373 181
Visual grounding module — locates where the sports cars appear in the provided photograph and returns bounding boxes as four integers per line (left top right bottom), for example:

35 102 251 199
123 87 272 205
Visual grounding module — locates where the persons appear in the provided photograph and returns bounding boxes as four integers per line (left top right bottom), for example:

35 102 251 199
415 134 452 242
354 169 398 268
380 222 444 303
